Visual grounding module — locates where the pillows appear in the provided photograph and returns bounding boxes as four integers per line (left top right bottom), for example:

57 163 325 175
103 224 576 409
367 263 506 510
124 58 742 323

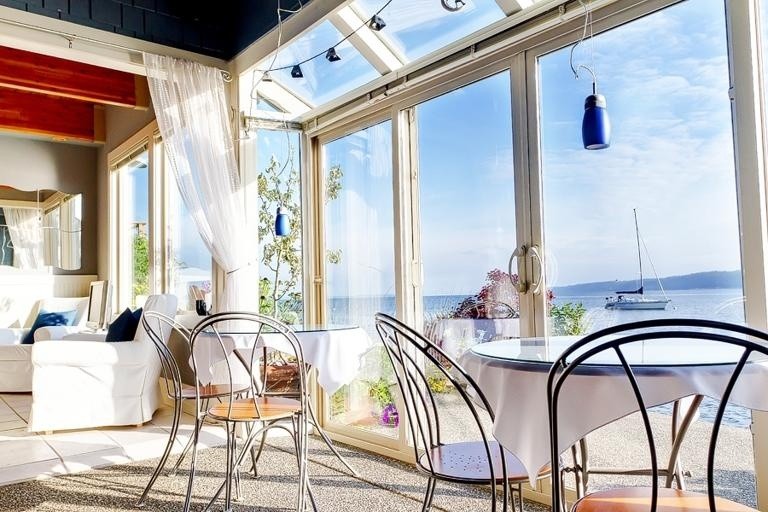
23 308 78 344
106 308 140 341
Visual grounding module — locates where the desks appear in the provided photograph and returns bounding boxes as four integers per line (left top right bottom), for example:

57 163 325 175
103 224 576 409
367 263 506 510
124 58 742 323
455 331 768 512
192 318 361 475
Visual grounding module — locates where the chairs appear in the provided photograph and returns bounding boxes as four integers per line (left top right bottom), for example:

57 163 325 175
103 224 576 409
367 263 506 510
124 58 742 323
30 294 177 436
541 317 768 512
376 313 557 512
138 310 261 504
1 296 91 394
180 311 318 512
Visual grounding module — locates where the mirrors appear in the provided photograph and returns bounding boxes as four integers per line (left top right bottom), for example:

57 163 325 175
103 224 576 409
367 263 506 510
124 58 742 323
2 184 84 271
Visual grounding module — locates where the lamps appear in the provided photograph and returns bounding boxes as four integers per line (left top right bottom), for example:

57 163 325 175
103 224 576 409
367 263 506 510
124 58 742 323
560 2 612 151
276 123 293 238
292 13 386 77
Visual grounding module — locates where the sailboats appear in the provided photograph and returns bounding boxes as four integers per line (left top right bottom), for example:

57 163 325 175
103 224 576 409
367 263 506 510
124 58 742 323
603 208 672 312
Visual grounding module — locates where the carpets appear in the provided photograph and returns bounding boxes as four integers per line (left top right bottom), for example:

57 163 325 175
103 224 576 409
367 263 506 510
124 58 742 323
0 431 547 510
